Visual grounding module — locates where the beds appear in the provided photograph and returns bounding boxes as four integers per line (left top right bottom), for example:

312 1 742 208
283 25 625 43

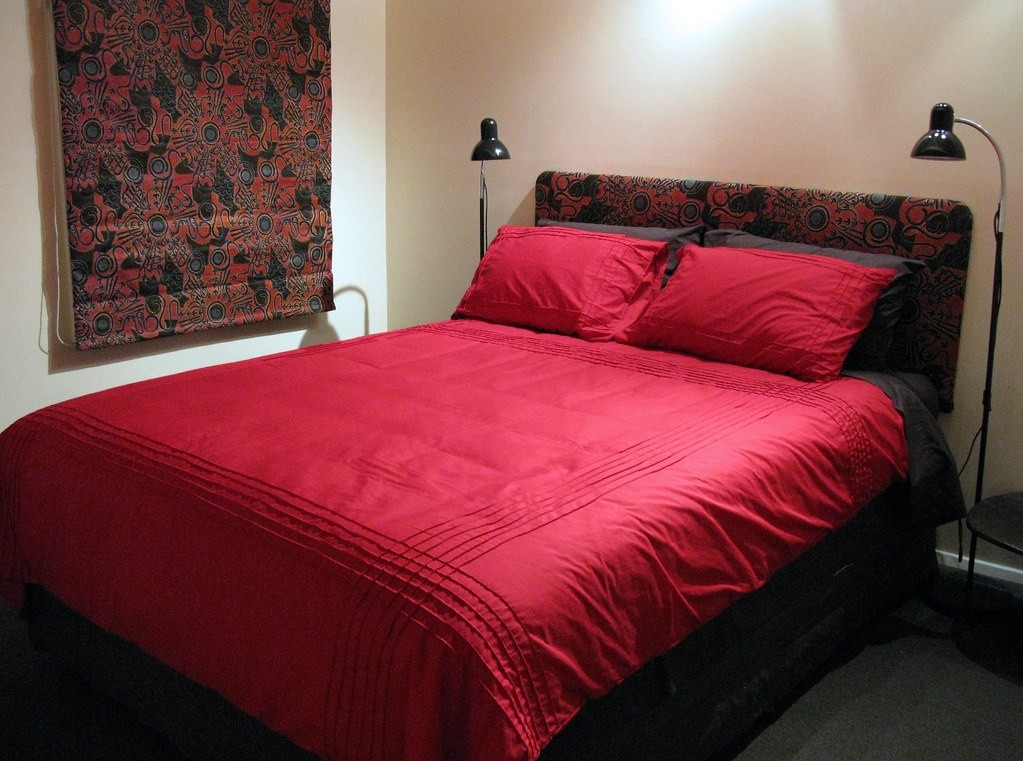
0 171 974 761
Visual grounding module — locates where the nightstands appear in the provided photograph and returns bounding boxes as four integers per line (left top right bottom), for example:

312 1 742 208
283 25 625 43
950 492 1023 687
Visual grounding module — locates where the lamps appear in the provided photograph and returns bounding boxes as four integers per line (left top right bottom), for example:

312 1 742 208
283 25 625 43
470 117 510 259
910 102 1018 648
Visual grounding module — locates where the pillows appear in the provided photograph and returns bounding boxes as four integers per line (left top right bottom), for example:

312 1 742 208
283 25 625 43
614 244 899 384
705 229 927 368
537 218 708 287
454 224 669 340
